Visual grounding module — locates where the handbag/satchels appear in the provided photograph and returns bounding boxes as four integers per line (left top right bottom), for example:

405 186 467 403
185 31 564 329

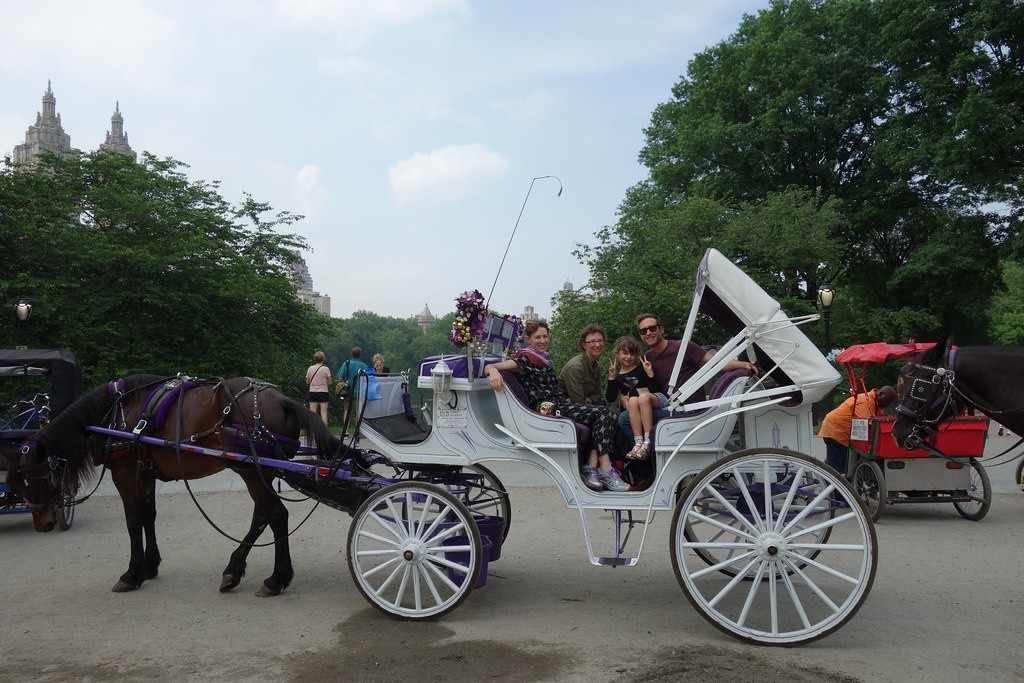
336 359 349 396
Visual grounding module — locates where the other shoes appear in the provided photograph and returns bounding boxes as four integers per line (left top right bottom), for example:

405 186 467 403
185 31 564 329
1006 431 1013 436
997 425 1004 436
635 442 653 460
343 433 349 439
629 480 649 491
625 445 640 459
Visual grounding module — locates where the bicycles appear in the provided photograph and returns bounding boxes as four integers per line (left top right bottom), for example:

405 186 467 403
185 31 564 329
1 391 52 431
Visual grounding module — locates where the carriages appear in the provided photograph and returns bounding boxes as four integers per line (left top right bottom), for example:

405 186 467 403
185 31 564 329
14 244 884 648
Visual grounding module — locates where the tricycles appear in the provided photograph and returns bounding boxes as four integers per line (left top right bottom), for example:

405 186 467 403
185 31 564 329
829 338 994 523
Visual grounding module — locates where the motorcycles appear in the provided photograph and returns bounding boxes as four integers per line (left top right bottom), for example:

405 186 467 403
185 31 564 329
0 348 81 533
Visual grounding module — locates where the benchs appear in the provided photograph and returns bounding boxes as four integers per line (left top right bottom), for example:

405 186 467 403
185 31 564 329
648 369 755 453
488 369 590 450
850 415 991 458
420 356 501 390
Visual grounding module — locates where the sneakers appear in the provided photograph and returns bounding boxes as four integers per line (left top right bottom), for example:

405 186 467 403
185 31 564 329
581 464 603 491
596 466 631 492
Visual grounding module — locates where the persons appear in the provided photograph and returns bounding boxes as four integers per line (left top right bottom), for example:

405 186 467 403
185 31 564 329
557 324 606 405
373 353 392 376
998 424 1012 436
484 320 630 492
818 386 896 506
618 314 759 491
337 347 368 440
306 351 332 426
605 336 669 460
898 358 913 399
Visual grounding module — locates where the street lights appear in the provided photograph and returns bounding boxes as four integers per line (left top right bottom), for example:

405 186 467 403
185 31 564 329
14 295 37 398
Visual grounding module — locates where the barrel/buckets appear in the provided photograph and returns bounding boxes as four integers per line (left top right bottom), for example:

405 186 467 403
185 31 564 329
469 491 506 562
442 534 494 590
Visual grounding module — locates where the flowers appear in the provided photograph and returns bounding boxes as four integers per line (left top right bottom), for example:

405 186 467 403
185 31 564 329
450 289 525 357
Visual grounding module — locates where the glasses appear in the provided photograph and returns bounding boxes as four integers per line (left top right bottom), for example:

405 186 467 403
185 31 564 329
585 340 604 344
526 318 546 324
639 325 659 335
